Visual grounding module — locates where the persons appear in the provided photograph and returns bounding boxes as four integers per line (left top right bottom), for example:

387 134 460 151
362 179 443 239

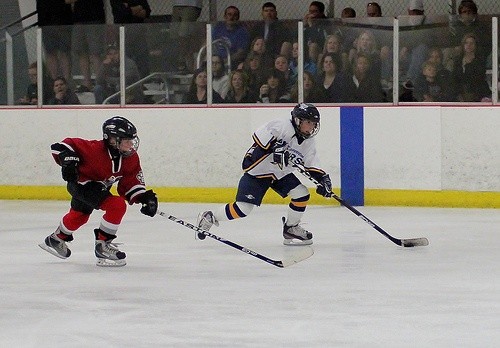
36 0 106 92
19 60 53 105
182 0 492 104
109 0 152 82
45 116 158 260
197 102 332 240
48 76 80 104
94 41 145 104
144 20 178 74
170 0 203 71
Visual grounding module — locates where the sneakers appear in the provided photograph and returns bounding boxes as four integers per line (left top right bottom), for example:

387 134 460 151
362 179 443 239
94 229 127 267
195 211 219 240
38 227 72 260
282 216 313 246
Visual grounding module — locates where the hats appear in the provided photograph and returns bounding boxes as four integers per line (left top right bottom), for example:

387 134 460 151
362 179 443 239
409 0 423 11
104 40 120 50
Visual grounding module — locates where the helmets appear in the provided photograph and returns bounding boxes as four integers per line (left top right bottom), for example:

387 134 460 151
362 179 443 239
291 103 320 139
103 116 140 155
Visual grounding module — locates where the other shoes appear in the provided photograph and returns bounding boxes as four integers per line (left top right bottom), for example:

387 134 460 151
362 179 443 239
75 85 90 92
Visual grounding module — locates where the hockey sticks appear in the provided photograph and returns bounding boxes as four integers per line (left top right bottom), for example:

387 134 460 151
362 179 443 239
142 203 314 268
287 159 429 248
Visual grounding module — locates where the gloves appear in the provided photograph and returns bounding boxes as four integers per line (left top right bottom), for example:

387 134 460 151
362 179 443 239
138 189 158 217
316 174 332 197
272 141 289 167
58 151 80 170
